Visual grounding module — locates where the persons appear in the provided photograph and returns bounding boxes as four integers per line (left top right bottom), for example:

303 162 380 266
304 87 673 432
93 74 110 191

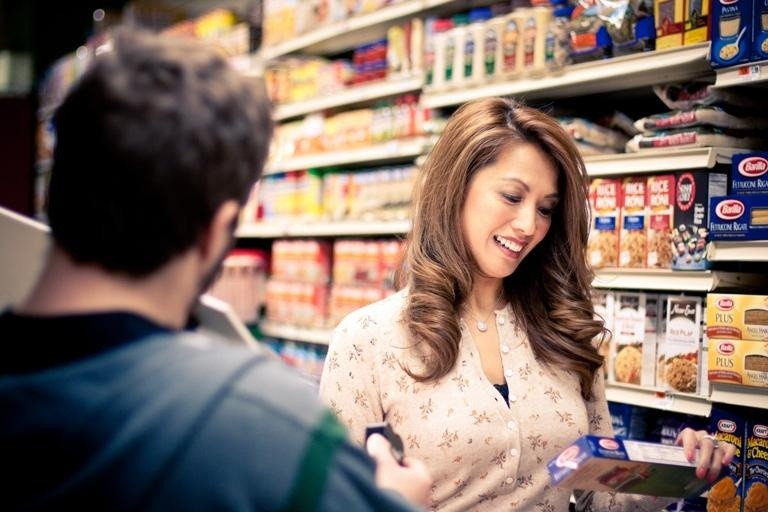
2 33 435 511
319 96 736 512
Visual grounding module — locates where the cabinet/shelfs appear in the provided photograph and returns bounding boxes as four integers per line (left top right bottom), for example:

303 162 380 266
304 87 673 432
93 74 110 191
35 4 768 512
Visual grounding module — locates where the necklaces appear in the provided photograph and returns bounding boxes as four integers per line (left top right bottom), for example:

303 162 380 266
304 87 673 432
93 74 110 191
460 289 505 332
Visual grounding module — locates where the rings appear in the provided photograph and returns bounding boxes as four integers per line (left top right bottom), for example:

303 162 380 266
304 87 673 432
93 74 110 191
703 434 720 448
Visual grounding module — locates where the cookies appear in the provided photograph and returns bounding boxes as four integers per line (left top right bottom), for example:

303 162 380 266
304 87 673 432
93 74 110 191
705 477 767 511
665 358 698 393
613 346 641 383
